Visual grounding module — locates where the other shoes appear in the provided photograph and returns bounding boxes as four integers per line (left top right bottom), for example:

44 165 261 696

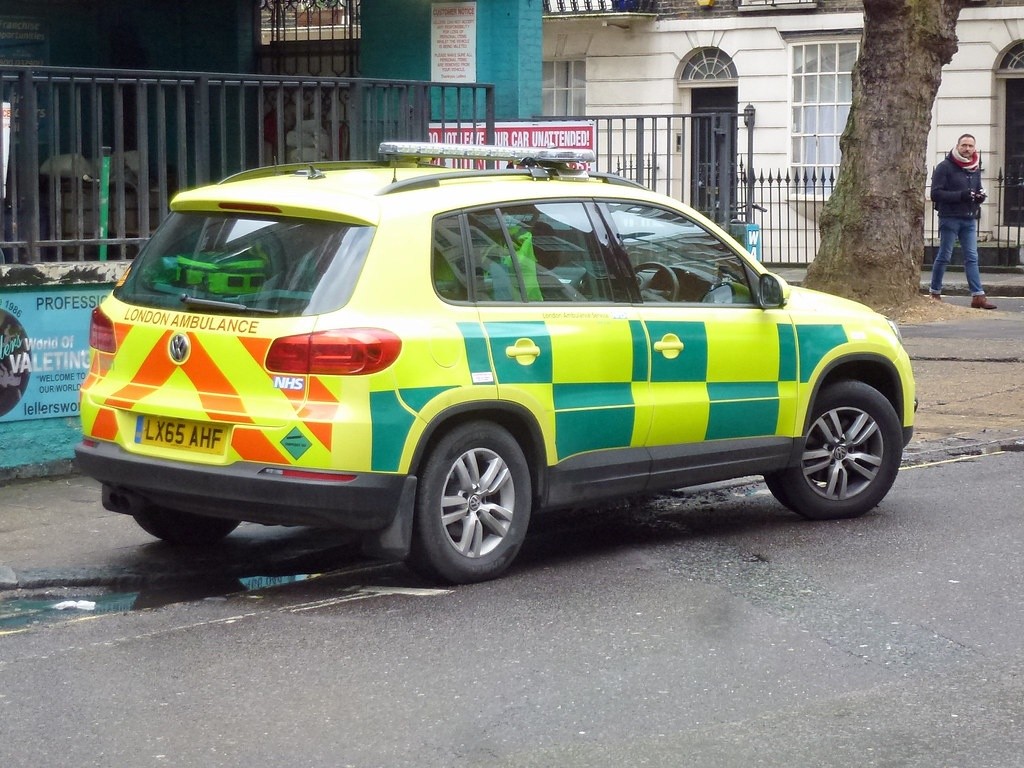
971 294 997 309
929 293 941 302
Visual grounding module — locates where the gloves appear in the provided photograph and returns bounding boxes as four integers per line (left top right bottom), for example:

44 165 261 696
977 191 988 204
961 190 976 203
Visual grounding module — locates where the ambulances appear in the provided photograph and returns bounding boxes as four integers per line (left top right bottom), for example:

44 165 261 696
77 141 919 583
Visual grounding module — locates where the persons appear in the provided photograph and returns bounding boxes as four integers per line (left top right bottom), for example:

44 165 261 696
930 134 997 309
532 222 588 302
